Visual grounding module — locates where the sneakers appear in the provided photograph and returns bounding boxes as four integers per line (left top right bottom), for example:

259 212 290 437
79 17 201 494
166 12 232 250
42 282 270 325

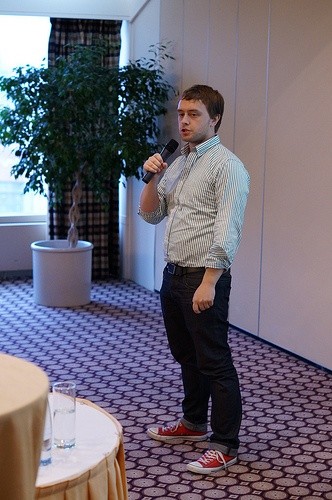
186 448 238 475
147 420 208 442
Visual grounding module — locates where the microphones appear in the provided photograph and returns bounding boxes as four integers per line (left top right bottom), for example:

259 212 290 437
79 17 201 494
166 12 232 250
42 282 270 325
142 138 179 184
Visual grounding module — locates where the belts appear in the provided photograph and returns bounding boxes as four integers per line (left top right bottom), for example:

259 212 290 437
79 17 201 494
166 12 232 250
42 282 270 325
166 263 232 274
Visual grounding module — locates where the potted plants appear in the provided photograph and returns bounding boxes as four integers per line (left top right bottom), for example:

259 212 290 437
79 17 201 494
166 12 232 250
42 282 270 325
0 37 180 307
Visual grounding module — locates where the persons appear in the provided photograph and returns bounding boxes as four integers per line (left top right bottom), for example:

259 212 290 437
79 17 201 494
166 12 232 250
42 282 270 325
137 85 250 474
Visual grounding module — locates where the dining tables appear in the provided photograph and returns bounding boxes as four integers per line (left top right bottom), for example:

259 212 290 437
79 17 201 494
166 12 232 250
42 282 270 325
39 393 128 500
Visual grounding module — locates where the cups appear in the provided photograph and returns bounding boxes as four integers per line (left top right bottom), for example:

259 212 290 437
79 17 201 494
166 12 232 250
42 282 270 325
52 382 76 448
40 399 52 466
